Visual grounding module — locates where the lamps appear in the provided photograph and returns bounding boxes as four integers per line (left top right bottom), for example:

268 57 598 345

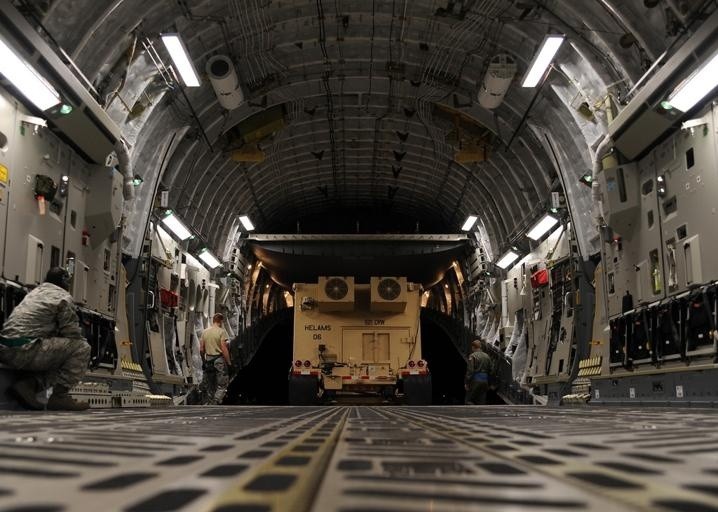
237 214 256 231
519 33 567 88
160 32 205 89
526 212 558 241
160 207 195 242
665 48 718 114
461 214 480 231
197 247 221 270
494 248 521 269
0 26 72 116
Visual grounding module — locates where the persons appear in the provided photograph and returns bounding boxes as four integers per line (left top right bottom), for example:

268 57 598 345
0 265 92 411
462 340 493 405
199 313 233 406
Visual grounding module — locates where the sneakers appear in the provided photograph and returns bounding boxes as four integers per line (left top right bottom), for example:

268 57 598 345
46 386 90 411
10 378 45 410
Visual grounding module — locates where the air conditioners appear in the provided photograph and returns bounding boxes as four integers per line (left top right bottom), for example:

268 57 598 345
370 275 406 314
316 275 354 312
